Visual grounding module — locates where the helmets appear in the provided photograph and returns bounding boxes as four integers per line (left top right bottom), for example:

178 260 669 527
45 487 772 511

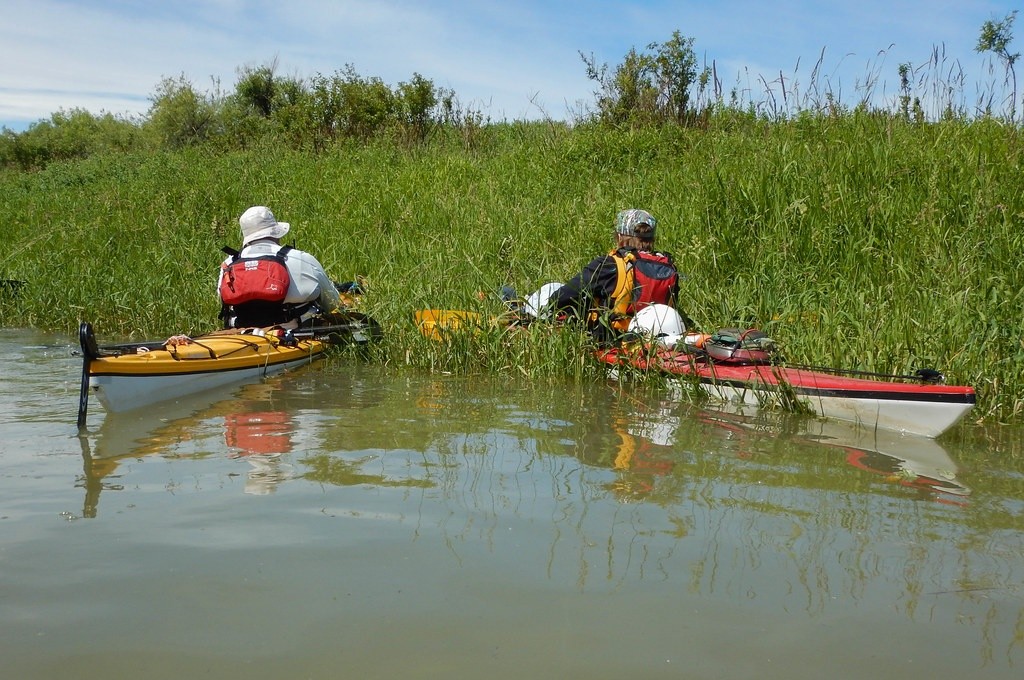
621 303 686 353
614 209 656 237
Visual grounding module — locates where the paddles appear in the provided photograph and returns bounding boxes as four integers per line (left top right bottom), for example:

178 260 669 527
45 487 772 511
97 311 385 351
413 307 528 344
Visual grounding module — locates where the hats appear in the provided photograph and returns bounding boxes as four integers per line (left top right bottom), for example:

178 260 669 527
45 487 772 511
239 206 290 247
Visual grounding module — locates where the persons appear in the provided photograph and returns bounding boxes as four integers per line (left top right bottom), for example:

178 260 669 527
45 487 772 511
217 206 369 334
502 209 680 350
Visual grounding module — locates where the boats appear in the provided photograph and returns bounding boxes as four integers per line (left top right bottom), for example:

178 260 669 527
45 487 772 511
83 354 338 515
603 386 973 508
413 305 975 440
76 275 367 416
